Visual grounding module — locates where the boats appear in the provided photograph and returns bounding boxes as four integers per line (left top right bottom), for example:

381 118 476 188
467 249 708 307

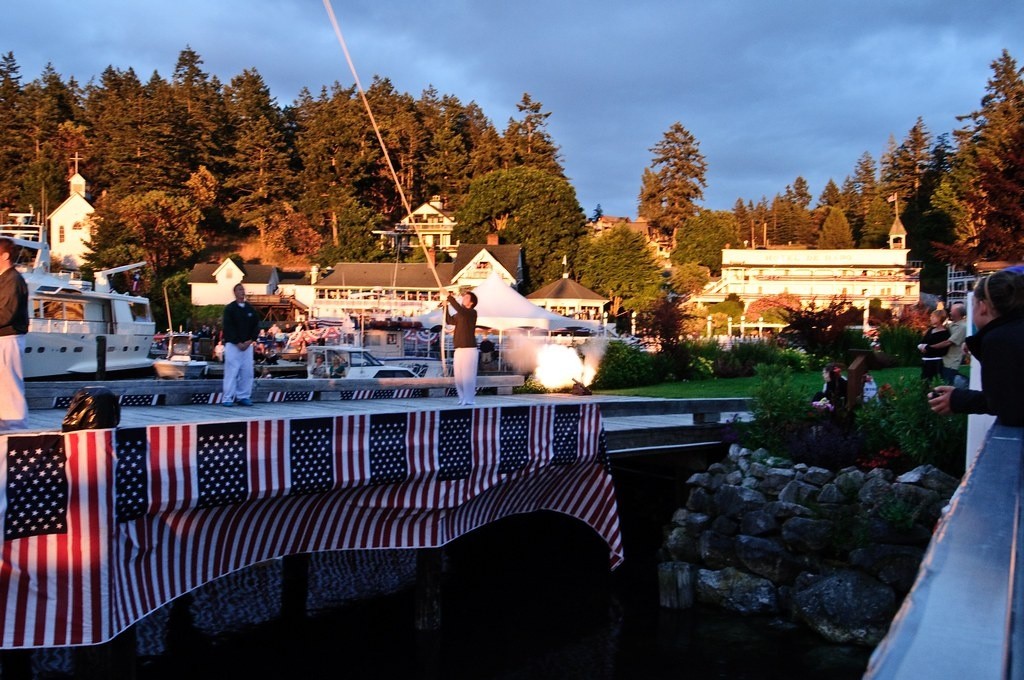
1 205 159 381
154 285 208 380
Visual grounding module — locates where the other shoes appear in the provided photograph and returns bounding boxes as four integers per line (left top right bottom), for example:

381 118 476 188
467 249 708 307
222 401 233 407
238 399 253 406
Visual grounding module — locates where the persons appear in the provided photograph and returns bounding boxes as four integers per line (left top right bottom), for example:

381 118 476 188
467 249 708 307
812 363 848 409
309 356 345 379
0 237 29 431
221 284 260 407
927 270 1024 426
442 291 479 405
258 367 272 380
917 304 966 385
157 321 325 361
480 336 495 362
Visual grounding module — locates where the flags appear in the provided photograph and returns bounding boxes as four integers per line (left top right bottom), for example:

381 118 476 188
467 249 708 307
132 270 141 293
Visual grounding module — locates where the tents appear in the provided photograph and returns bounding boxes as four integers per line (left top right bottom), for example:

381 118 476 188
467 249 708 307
396 271 600 371
